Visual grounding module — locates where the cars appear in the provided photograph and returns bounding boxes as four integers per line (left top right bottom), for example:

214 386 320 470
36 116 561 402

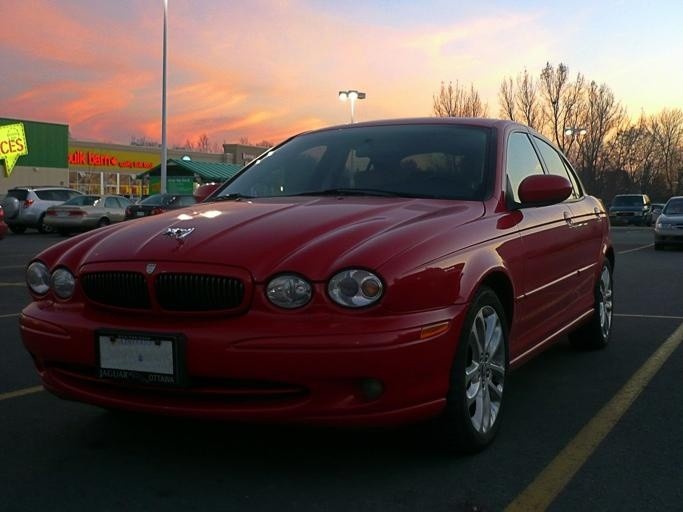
0 183 205 236
13 114 616 463
606 190 683 251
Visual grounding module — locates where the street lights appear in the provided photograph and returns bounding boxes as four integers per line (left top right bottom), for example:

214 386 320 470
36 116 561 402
336 88 366 121
563 125 586 173
